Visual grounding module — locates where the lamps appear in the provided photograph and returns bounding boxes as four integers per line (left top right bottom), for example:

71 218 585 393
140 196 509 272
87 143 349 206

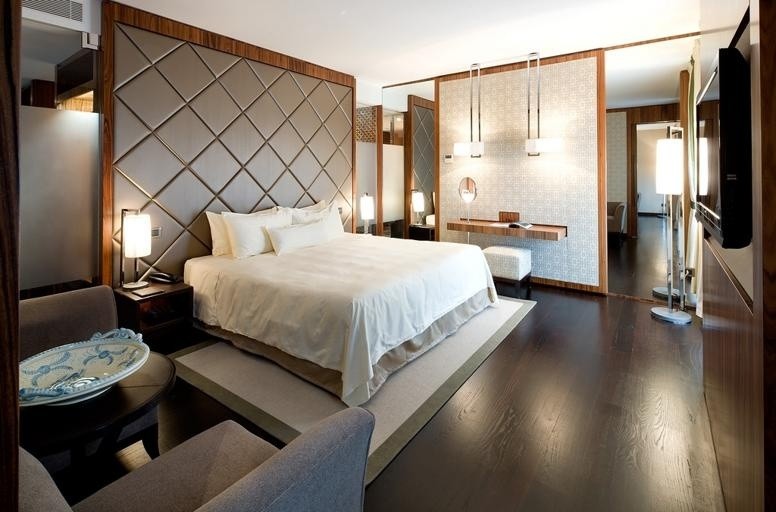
467 64 484 159
412 192 425 226
652 193 680 299
651 137 692 326
524 52 544 157
119 207 152 291
360 192 374 234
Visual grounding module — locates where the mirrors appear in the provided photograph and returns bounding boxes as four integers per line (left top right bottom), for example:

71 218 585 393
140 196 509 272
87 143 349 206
459 177 477 222
382 77 435 241
605 34 701 307
20 21 101 114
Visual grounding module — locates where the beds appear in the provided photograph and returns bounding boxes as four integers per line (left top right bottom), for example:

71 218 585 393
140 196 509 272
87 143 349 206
183 233 499 409
426 214 435 227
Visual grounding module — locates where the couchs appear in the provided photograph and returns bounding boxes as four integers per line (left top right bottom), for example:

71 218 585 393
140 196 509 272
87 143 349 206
608 202 626 247
18 406 375 512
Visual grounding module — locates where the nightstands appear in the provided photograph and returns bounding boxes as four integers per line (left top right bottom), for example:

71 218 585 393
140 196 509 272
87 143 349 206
113 280 194 355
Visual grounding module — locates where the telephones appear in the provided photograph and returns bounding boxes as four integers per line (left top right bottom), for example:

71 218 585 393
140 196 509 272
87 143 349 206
149 272 180 284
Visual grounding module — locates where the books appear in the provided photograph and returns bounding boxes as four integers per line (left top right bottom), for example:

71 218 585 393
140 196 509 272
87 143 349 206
490 219 532 233
132 287 165 297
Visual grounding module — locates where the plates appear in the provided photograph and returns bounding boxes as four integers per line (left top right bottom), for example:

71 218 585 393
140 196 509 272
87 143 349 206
18 328 151 409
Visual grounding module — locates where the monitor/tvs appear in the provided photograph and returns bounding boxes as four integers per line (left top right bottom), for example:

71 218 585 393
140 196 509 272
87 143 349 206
692 47 751 250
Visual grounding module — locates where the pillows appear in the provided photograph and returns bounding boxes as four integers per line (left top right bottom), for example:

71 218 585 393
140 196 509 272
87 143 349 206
433 191 436 214
205 200 345 259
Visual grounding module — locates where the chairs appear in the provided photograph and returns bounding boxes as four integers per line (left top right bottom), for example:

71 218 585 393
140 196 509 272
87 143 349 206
18 283 120 363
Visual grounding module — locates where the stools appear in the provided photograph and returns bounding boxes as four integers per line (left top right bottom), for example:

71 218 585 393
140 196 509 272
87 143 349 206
482 245 531 295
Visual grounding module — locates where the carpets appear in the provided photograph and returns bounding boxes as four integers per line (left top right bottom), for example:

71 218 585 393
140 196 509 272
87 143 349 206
166 294 538 488
